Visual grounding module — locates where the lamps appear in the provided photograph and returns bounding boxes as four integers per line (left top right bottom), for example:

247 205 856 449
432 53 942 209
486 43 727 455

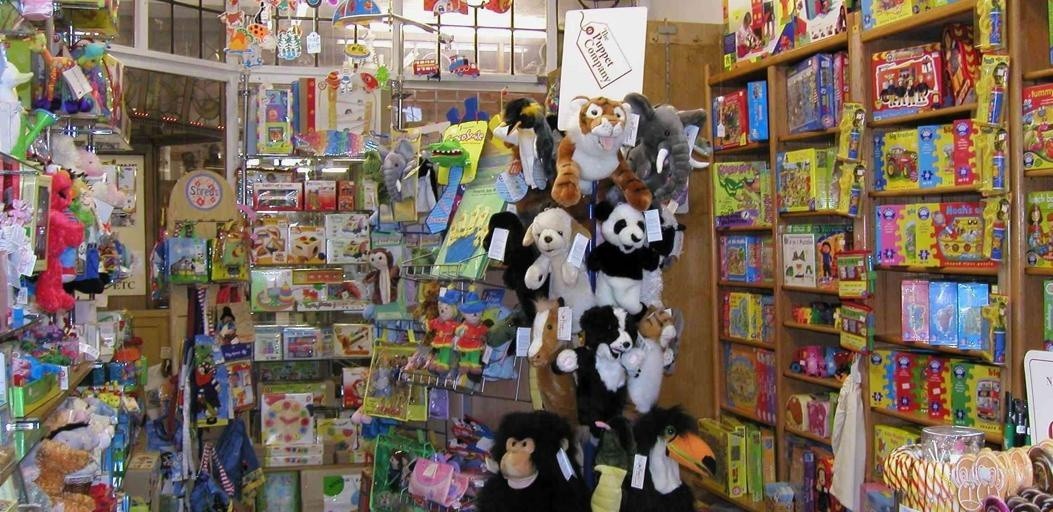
402 50 417 69
337 37 530 53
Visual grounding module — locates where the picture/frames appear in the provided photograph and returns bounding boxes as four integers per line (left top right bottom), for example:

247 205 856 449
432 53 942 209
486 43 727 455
127 309 170 368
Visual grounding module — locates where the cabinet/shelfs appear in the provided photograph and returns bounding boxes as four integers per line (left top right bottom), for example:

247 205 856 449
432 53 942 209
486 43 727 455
242 151 374 470
0 152 136 512
704 56 779 512
856 2 1024 477
1010 0 1053 398
778 28 869 483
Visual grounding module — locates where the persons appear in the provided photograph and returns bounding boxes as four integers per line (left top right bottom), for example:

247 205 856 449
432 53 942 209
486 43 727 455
735 14 762 58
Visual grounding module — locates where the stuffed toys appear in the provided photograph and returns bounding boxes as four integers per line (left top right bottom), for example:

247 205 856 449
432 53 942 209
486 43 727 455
0 0 511 512
348 91 716 512
